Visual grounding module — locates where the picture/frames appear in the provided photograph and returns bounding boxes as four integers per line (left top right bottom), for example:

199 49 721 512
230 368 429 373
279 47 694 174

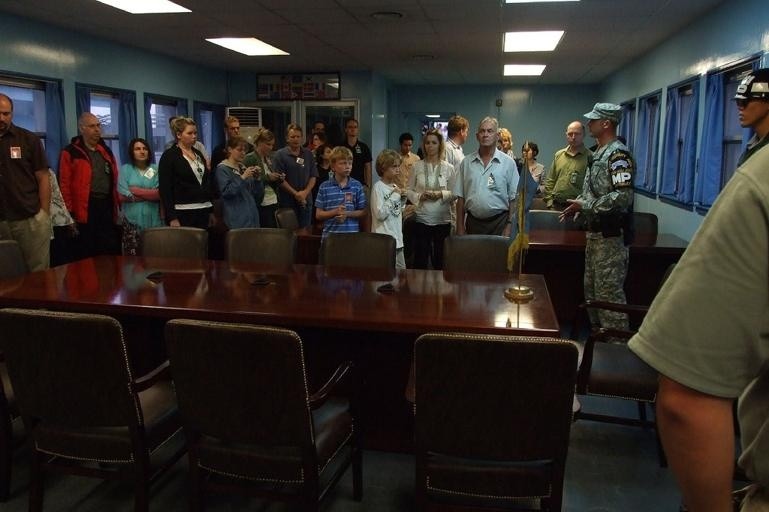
525 228 689 306
0 256 562 334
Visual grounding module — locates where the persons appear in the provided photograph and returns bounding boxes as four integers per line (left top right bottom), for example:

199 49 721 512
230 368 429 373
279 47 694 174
407 130 457 270
302 121 329 165
164 118 212 176
270 123 318 232
215 137 264 231
454 117 519 236
155 116 217 237
370 149 408 270
626 145 767 512
0 93 51 282
522 142 544 198
58 113 124 260
393 132 421 213
241 127 285 229
496 128 520 175
732 69 768 169
443 117 469 235
315 146 368 265
545 121 594 211
555 104 635 347
211 117 255 259
311 143 336 236
339 118 372 233
118 138 158 256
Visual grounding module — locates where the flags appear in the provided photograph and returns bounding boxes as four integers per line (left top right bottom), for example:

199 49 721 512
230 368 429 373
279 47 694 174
508 157 538 272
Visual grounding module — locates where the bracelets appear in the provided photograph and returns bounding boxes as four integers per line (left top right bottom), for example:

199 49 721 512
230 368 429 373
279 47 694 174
363 181 370 188
506 221 512 224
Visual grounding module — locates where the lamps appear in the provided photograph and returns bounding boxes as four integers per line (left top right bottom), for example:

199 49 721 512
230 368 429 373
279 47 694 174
227 227 290 262
275 207 299 231
165 320 365 512
325 232 396 265
444 236 512 272
0 309 182 512
529 209 566 234
406 332 581 512
600 212 659 241
0 240 29 279
567 299 669 468
143 227 208 257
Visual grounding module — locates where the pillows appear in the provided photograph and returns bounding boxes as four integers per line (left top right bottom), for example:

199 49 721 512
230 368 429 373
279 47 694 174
730 68 769 100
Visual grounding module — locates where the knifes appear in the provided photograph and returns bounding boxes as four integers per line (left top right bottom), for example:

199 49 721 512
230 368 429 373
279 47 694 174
225 106 262 147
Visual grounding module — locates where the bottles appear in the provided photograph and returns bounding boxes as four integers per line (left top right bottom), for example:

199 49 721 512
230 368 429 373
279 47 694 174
583 103 622 124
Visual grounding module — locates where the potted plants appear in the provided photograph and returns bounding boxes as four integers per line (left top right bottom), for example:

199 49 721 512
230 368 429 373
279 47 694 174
256 73 342 101
